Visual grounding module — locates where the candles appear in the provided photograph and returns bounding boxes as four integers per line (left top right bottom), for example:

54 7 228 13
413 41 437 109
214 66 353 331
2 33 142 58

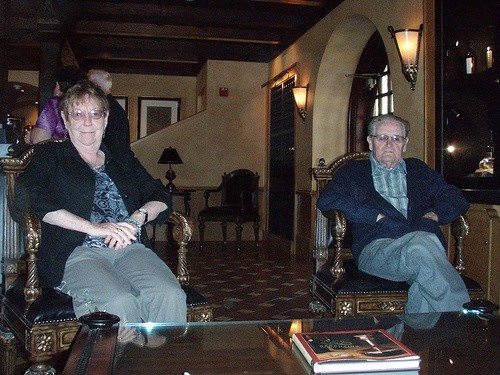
486 46 492 68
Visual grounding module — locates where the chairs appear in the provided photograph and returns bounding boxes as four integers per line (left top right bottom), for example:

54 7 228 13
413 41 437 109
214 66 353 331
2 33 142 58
198 169 260 248
0 159 212 375
310 151 470 320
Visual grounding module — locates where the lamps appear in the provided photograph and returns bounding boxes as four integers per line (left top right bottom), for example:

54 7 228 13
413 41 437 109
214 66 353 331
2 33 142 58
157 145 183 190
387 24 424 91
292 87 307 122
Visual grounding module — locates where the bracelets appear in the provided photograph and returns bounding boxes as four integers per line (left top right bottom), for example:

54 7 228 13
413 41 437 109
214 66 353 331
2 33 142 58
124 216 141 227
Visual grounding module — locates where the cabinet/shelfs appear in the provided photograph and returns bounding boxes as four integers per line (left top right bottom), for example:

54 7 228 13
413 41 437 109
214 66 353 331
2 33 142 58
447 204 500 304
422 0 500 204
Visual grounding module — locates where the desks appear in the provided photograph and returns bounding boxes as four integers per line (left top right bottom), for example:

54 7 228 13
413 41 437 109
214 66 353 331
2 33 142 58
56 310 500 375
152 186 221 245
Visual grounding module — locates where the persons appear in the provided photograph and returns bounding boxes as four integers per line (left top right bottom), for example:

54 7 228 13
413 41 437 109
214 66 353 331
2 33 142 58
316 114 471 313
12 68 187 325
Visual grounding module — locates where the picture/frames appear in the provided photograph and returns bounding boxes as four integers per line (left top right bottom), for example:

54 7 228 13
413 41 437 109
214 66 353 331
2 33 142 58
138 96 181 142
113 95 128 117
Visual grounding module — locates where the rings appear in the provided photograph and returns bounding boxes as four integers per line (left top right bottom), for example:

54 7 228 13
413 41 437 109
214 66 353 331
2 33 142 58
130 222 133 225
119 229 122 232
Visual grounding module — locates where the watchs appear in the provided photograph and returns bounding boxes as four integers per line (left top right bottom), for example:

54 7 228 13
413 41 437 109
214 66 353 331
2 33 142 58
134 208 148 225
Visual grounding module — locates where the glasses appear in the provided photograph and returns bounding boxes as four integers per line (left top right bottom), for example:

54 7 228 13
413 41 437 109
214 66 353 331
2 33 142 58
370 133 405 144
68 109 105 121
